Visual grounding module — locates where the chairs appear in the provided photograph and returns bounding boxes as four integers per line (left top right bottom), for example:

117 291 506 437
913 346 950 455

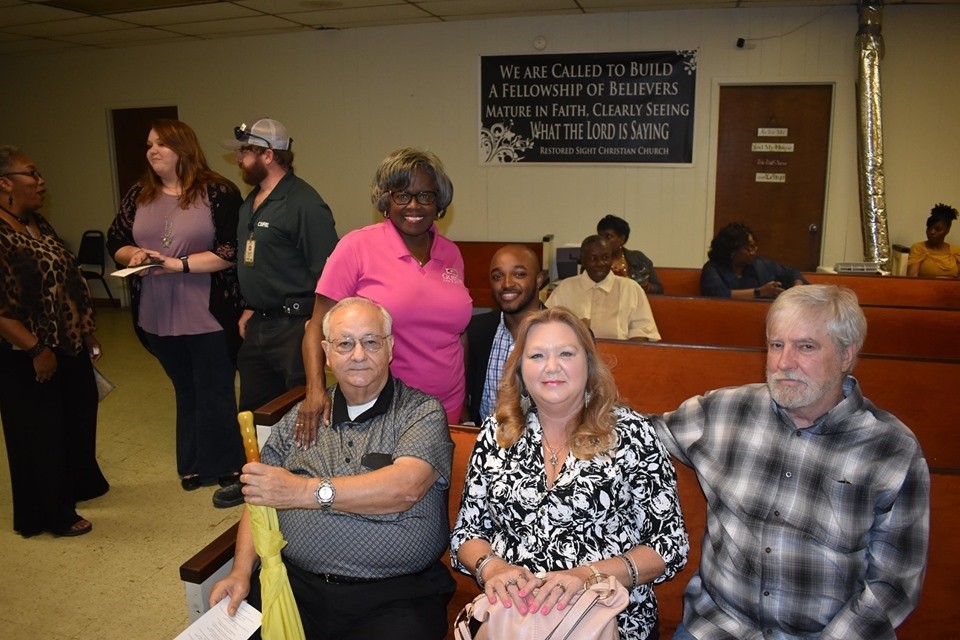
78 231 115 306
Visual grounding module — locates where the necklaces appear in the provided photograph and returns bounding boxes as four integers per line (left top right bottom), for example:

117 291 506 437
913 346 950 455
538 425 573 472
405 230 431 268
610 251 628 273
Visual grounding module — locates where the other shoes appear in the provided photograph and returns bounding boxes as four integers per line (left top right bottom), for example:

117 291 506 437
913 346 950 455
218 472 240 487
181 474 199 490
212 482 245 508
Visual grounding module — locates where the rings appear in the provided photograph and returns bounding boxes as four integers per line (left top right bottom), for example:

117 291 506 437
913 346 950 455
516 574 525 581
535 571 549 585
296 423 303 428
555 583 565 592
505 578 516 590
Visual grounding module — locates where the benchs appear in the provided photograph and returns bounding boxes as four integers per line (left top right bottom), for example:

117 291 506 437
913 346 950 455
179 242 960 640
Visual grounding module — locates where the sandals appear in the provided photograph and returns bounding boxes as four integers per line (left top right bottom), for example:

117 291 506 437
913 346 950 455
20 515 92 537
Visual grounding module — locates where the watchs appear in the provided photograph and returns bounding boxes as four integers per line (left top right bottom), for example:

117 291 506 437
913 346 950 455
313 475 336 511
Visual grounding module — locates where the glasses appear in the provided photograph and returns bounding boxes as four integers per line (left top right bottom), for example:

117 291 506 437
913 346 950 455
3 171 42 179
235 125 272 149
388 189 437 205
329 334 389 353
744 244 759 253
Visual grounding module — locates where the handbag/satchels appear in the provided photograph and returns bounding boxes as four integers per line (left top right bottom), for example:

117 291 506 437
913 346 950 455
454 576 629 640
93 365 114 401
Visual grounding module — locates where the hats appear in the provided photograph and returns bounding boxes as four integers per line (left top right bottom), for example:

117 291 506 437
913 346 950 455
248 119 290 150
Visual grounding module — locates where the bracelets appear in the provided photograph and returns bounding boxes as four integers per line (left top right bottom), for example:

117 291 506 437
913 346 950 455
755 287 760 297
616 553 639 591
581 561 601 583
26 340 45 359
179 254 191 275
475 553 507 593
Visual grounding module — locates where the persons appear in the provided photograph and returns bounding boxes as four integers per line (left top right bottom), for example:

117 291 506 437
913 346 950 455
597 214 664 296
292 145 475 451
104 119 246 492
699 220 810 300
544 234 663 341
643 285 930 640
907 202 960 280
211 116 342 508
0 143 110 539
449 306 691 640
464 243 597 431
206 296 454 640
